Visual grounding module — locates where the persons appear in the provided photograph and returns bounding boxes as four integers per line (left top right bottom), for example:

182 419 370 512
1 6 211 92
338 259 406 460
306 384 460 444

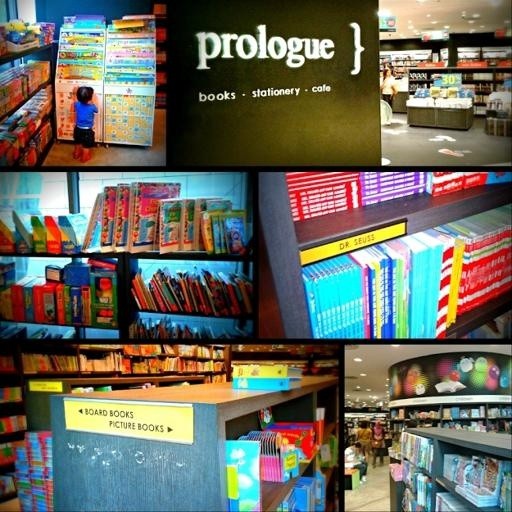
344 442 368 485
371 420 386 468
70 87 98 163
356 420 372 463
379 68 398 108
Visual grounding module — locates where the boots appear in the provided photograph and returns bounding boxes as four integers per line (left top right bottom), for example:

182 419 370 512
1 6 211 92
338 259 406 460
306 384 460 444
80 149 92 163
72 145 82 159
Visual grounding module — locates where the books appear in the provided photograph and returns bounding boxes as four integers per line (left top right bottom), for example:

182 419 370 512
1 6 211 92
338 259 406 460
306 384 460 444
402 459 432 512
401 432 434 475
443 453 512 512
0 181 252 252
20 344 228 385
287 172 512 222
129 258 254 339
301 203 511 339
232 364 303 391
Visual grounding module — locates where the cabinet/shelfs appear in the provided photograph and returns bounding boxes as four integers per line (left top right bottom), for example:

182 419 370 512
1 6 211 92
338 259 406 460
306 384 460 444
0 168 257 339
0 344 233 505
454 38 511 114
0 20 57 170
51 376 340 512
258 167 511 337
380 38 452 94
386 394 512 437
407 94 474 129
386 428 511 512
